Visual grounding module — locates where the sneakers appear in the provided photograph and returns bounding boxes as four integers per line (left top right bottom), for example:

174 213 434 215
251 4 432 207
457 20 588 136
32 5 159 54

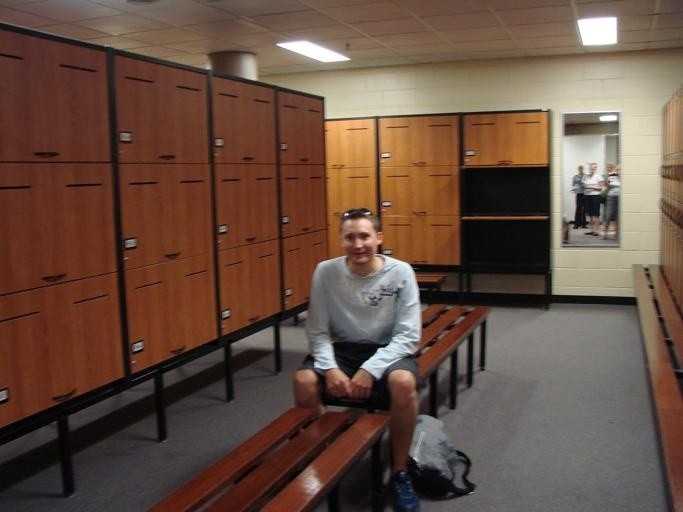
391 470 420 511
572 224 608 241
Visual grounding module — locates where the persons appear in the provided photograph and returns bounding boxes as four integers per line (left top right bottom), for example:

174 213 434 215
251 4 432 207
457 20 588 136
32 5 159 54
294 208 423 512
569 161 620 240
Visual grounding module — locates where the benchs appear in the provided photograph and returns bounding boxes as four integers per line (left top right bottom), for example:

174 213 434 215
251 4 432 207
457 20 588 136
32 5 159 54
415 273 448 296
630 263 683 508
286 302 491 418
147 407 392 512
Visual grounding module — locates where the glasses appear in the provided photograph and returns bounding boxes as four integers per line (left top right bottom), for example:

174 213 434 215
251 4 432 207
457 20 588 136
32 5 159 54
342 207 374 220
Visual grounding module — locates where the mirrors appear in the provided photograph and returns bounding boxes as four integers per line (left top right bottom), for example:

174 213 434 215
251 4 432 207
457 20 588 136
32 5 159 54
560 109 623 249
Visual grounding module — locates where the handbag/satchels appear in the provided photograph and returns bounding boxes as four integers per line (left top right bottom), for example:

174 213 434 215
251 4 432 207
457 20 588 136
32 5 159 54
407 414 456 498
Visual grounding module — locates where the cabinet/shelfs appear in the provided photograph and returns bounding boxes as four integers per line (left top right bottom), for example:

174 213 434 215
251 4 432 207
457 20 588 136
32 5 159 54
659 84 683 317
